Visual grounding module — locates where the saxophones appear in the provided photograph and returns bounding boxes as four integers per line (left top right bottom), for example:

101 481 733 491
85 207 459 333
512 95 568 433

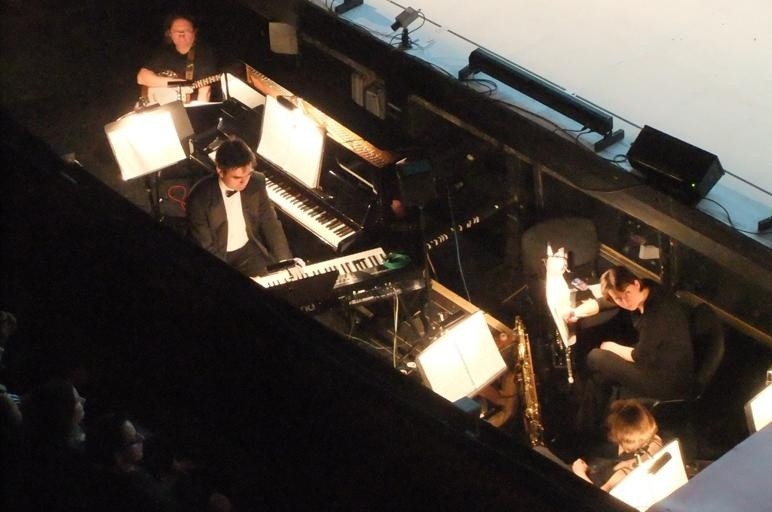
512 316 545 448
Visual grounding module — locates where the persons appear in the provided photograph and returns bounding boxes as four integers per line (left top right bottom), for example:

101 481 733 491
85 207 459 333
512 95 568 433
0 379 230 512
185 139 292 277
564 266 694 400
136 15 223 134
549 398 663 485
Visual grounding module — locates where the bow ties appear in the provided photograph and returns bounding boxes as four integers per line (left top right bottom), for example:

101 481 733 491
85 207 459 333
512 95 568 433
226 190 237 197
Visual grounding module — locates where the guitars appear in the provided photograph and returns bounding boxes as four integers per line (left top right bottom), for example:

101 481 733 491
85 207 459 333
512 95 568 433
141 66 244 108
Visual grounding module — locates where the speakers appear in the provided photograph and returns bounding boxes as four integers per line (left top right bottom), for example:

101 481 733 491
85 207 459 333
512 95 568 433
627 125 723 199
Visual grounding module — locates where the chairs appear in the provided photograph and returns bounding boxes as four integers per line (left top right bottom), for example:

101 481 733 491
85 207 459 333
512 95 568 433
612 302 724 415
523 216 621 332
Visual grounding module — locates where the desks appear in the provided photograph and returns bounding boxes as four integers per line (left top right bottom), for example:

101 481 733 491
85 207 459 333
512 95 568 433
564 275 620 330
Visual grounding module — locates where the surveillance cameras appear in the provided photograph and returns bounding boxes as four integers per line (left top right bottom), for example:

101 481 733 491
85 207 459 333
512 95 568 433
389 6 419 32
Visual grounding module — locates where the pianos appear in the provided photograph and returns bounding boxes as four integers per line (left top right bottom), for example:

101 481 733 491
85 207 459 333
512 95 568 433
250 247 394 308
190 65 401 257
